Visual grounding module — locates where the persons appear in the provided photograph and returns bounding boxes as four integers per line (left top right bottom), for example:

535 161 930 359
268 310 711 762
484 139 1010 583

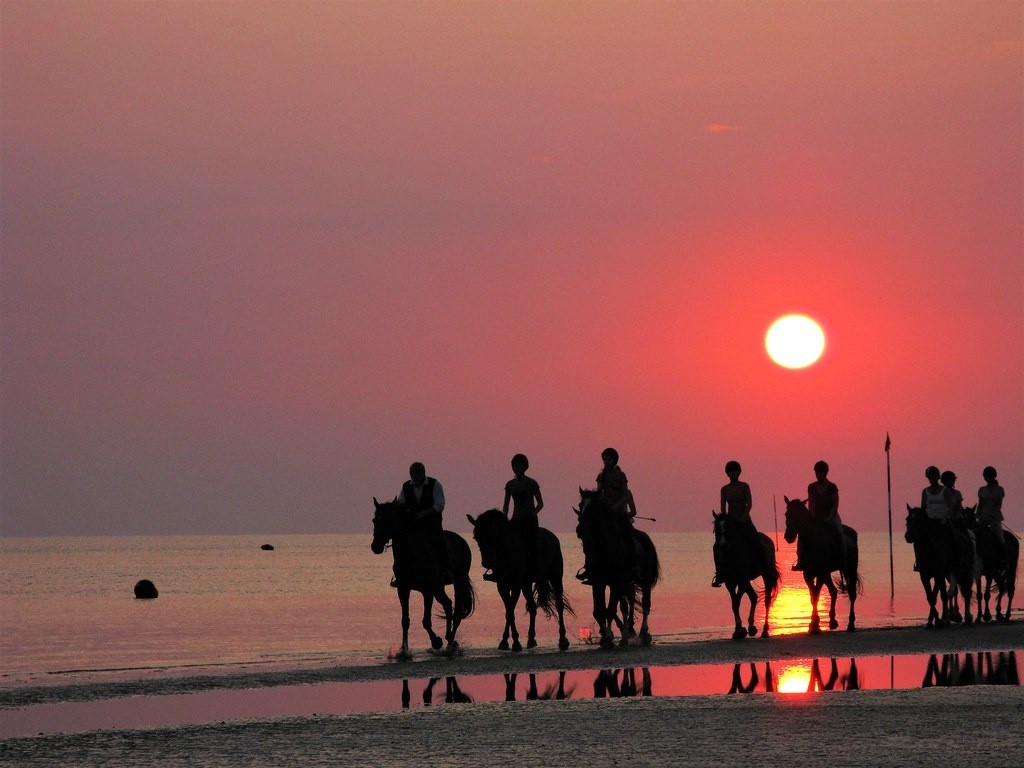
791 460 848 572
976 467 1010 569
483 454 543 583
712 460 757 587
390 462 446 587
575 449 637 586
913 464 974 570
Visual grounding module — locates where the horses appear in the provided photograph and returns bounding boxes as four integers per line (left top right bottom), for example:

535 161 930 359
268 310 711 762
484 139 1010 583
711 510 783 640
782 495 864 636
371 495 478 658
576 486 661 650
904 502 1019 630
465 509 577 652
